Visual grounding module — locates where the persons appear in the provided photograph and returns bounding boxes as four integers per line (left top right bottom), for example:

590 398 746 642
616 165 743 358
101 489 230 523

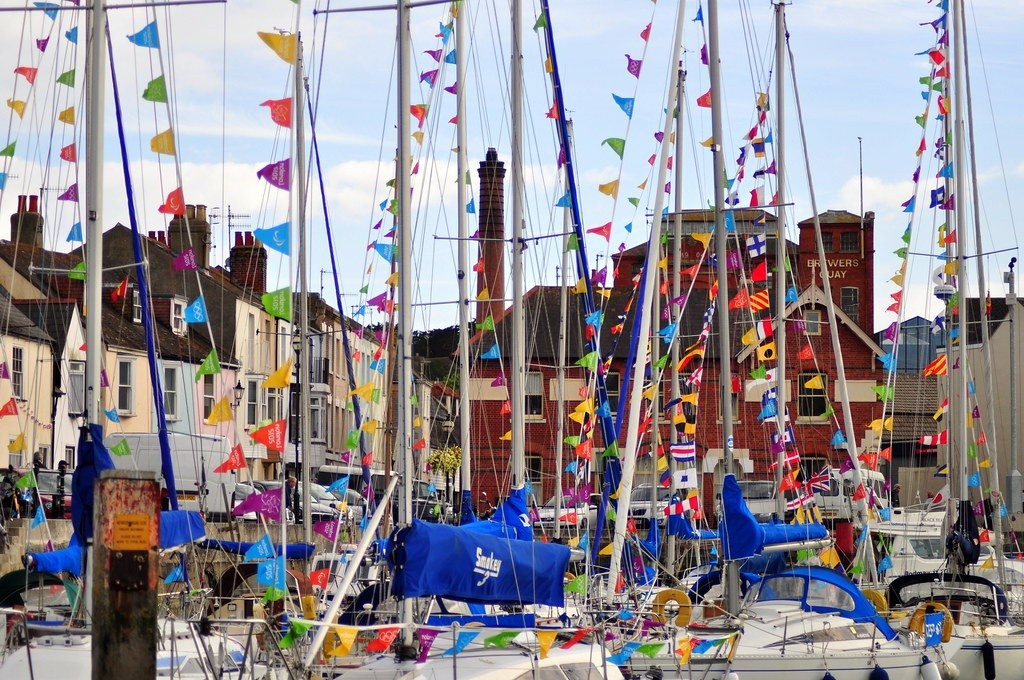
31 451 48 518
285 477 297 508
52 460 70 518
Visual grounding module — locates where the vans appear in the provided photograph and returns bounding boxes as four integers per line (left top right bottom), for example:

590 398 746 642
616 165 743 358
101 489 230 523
100 431 235 519
812 468 890 530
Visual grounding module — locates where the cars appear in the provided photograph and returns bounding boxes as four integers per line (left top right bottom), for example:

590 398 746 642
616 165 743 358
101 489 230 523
236 481 367 526
0 468 75 519
531 493 605 529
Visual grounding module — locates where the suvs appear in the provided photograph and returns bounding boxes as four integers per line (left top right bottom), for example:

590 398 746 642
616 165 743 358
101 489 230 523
737 481 797 523
628 483 680 530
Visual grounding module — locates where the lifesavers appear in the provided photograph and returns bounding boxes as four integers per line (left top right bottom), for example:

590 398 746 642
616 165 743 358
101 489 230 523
909 602 953 642
861 589 888 617
652 590 691 626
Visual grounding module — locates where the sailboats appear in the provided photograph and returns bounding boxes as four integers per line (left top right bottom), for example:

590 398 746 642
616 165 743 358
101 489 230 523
0 0 1024 680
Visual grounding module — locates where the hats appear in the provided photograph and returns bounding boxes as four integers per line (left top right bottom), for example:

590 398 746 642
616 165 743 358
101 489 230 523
59 460 70 466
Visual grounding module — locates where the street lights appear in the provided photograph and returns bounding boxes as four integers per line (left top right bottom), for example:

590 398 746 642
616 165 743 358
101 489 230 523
442 414 454 506
291 327 303 524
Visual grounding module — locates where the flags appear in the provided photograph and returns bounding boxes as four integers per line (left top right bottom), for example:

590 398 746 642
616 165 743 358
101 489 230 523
414 629 438 663
605 630 741 666
327 627 359 656
922 355 947 379
919 429 947 445
537 631 558 660
440 632 481 656
278 618 314 648
366 627 401 652
930 185 945 208
559 630 592 649
664 188 735 516
927 484 951 509
933 397 947 422
929 309 945 334
740 93 831 496
484 632 521 650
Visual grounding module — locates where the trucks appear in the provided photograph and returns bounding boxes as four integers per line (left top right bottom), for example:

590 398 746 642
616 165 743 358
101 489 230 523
317 465 446 525
